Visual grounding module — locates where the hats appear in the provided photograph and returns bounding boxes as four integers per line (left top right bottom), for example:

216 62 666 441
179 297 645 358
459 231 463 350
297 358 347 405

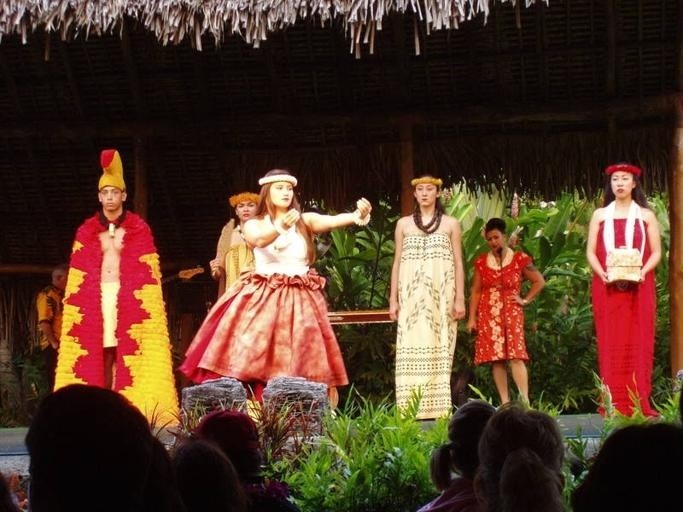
97 149 128 193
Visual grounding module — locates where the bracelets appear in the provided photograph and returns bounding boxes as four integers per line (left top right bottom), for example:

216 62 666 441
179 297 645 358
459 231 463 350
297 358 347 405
274 221 289 237
352 208 370 226
523 299 529 305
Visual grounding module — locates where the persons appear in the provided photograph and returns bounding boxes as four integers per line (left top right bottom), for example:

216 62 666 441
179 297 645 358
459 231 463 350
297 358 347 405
585 164 661 416
36 264 68 389
209 193 264 299
388 173 466 421
180 168 373 420
468 218 546 406
53 150 179 427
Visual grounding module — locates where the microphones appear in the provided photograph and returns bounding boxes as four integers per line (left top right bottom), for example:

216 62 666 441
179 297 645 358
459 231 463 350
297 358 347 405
496 246 504 254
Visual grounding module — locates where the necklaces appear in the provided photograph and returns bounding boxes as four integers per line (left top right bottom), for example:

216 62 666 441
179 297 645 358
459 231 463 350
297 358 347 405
416 209 441 234
490 246 511 267
413 208 438 230
